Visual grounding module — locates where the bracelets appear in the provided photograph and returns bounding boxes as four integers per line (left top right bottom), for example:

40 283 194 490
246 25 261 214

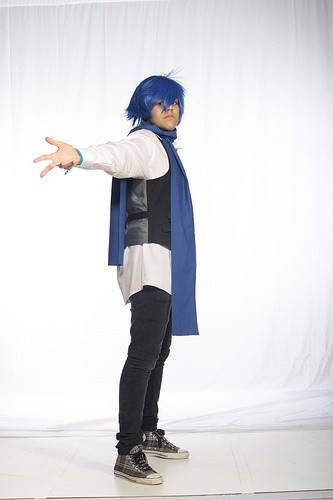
64 148 83 174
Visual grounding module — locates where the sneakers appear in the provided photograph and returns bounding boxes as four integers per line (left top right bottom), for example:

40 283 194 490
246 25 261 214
113 445 162 484
143 430 188 459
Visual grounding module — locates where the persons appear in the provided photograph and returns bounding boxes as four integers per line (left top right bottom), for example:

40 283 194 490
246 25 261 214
34 70 200 484
